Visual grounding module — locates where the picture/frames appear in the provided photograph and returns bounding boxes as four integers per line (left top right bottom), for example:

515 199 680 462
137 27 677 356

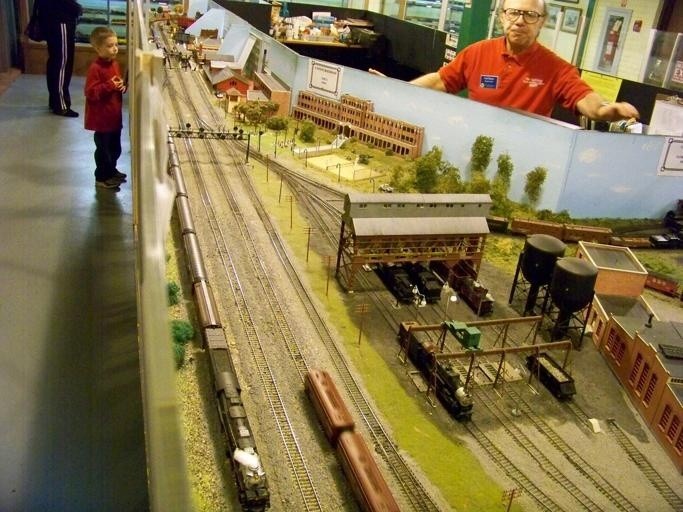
544 0 583 35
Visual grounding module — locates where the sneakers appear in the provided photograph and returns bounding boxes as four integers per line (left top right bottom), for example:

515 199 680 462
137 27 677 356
48 105 78 118
94 177 119 189
111 170 126 181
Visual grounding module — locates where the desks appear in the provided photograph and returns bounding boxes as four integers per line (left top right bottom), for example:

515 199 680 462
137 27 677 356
275 17 365 63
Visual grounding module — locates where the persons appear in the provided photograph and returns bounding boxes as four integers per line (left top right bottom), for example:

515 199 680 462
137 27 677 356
34 0 84 117
85 27 128 188
368 0 640 121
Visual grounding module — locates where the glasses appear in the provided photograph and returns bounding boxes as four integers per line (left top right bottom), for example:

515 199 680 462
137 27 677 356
503 8 545 25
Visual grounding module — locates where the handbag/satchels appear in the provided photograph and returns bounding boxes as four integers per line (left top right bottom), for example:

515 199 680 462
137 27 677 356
24 15 45 43
